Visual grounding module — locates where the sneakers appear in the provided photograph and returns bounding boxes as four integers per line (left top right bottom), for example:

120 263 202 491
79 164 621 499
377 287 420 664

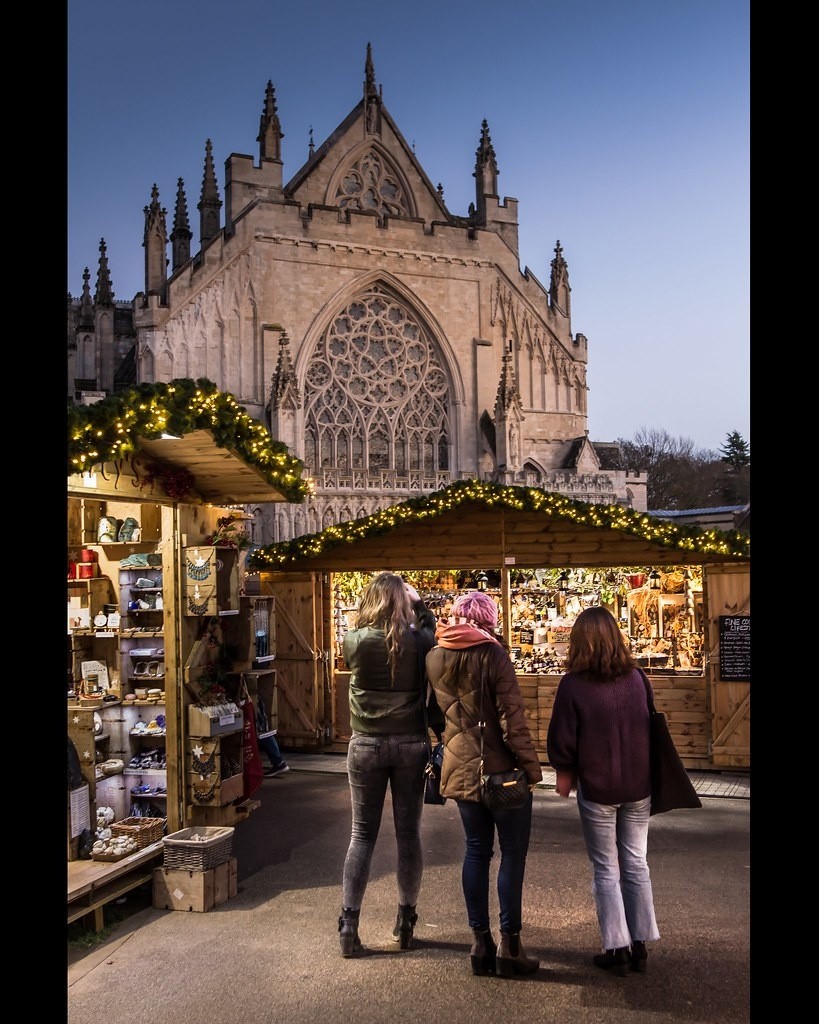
263 761 290 776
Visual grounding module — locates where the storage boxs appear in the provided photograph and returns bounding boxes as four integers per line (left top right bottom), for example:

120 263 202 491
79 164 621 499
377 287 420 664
152 856 238 913
68 779 91 862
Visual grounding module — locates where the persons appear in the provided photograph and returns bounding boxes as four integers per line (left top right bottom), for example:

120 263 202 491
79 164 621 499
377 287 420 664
260 736 290 777
423 591 543 979
336 573 440 959
545 608 703 976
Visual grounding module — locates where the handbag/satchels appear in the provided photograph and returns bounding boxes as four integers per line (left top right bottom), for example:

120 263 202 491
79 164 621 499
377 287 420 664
480 770 529 810
423 742 448 805
635 665 702 816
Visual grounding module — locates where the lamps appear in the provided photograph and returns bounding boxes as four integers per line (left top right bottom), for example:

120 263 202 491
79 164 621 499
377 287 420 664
161 428 184 440
477 569 489 592
650 566 662 589
558 567 571 590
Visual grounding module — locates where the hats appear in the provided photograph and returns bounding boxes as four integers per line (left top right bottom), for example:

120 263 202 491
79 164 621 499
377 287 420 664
451 592 498 628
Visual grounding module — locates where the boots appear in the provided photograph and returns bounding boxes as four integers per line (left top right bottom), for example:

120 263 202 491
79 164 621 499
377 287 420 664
392 902 418 948
338 908 362 957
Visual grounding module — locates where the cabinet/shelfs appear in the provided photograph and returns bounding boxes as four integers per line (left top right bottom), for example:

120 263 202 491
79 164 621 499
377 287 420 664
332 564 703 674
67 496 168 836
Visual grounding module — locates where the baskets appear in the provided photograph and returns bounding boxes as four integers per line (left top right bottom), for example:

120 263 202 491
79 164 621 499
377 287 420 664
162 826 235 871
89 817 164 861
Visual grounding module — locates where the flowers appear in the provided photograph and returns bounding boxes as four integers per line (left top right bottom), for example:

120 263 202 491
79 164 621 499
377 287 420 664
198 614 232 706
199 510 256 560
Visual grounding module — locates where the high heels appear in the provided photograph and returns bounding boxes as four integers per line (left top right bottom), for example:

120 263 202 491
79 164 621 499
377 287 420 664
495 929 539 977
471 929 496 976
594 940 647 973
